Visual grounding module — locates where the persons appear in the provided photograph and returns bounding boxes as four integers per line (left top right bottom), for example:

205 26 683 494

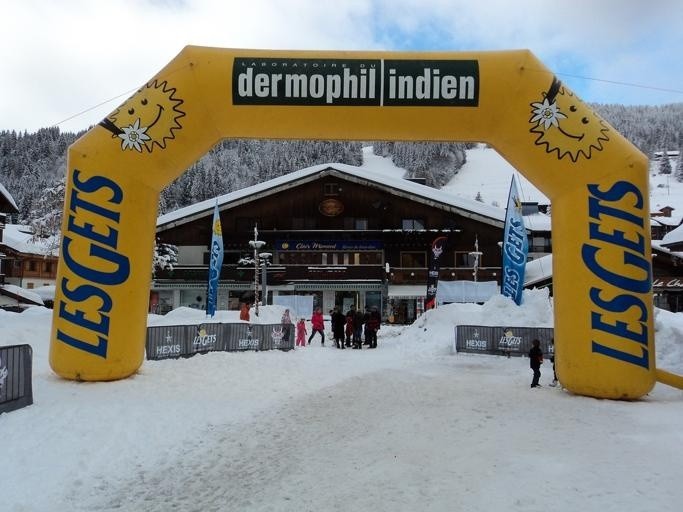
548 338 558 387
528 339 544 388
240 301 250 321
329 304 381 349
296 317 307 347
307 306 324 346
281 309 291 341
